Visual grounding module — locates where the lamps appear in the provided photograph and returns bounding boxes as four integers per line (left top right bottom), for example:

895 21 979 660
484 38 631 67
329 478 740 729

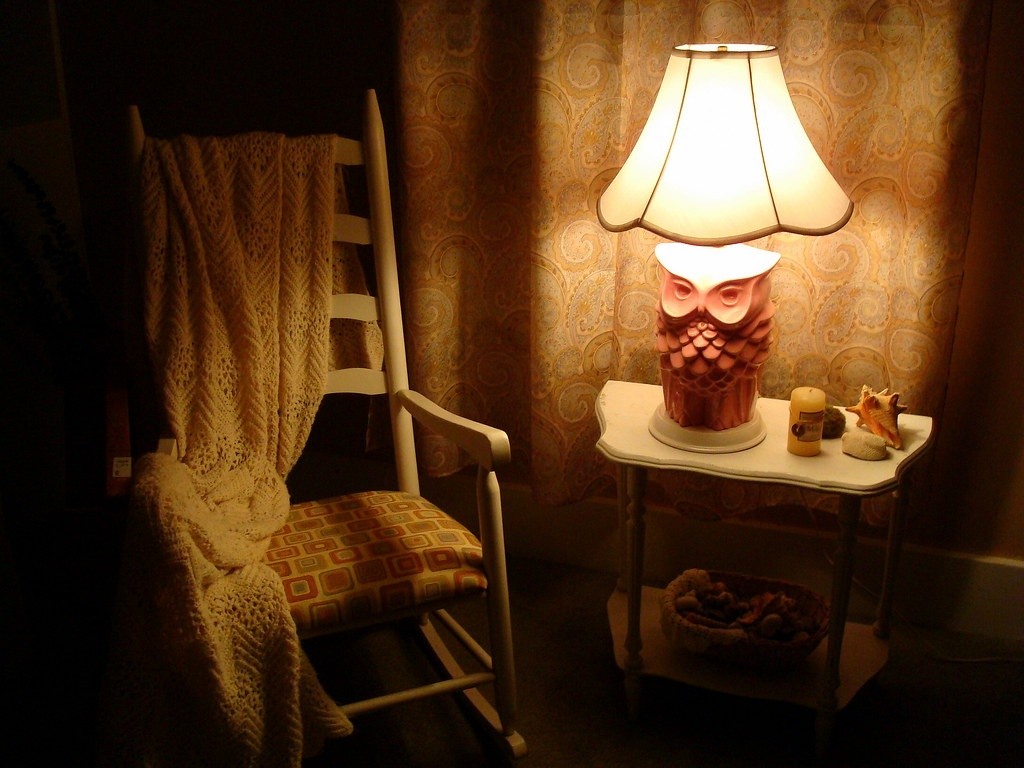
596 42 850 453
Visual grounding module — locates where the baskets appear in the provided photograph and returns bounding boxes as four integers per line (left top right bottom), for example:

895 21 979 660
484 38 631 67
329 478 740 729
663 567 833 671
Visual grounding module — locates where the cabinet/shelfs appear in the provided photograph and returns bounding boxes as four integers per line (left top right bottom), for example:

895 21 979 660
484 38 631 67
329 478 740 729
596 380 932 768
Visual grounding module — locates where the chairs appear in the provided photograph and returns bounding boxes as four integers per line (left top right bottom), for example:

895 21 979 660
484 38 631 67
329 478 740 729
104 89 552 768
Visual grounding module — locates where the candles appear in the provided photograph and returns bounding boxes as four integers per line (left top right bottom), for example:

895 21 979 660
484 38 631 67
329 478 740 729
788 385 826 457
